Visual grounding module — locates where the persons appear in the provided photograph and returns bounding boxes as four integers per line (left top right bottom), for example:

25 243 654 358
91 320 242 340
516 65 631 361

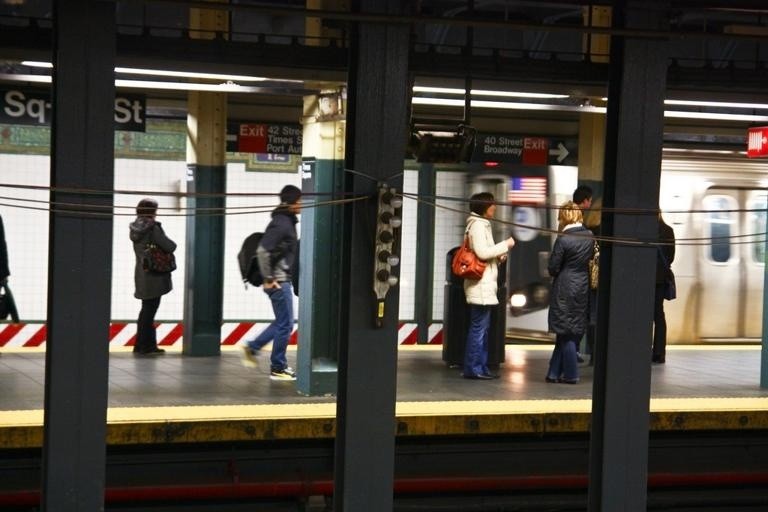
0 215 11 291
129 199 177 354
241 184 302 382
545 186 675 383
462 192 516 379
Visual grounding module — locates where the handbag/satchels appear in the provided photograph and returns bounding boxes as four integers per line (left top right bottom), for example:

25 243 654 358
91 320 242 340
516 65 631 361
664 267 678 301
588 259 601 290
141 221 177 276
450 219 488 281
235 231 268 290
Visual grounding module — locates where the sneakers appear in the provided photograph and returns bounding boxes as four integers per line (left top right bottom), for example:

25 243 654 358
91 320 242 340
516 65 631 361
131 346 167 357
545 375 578 385
240 343 261 371
269 366 298 382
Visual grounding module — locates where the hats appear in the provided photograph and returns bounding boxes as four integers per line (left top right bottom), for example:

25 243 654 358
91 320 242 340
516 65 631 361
278 185 303 205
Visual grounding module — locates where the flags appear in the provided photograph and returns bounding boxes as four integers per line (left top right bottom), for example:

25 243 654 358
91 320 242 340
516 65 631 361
508 177 548 207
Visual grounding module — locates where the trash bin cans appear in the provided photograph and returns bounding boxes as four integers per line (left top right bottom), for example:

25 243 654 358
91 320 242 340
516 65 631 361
442 246 507 372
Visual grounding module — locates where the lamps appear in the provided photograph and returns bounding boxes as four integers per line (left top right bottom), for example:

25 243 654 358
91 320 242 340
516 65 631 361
375 190 402 287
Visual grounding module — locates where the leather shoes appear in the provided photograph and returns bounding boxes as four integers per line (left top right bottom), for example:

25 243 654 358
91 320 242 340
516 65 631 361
465 370 494 381
